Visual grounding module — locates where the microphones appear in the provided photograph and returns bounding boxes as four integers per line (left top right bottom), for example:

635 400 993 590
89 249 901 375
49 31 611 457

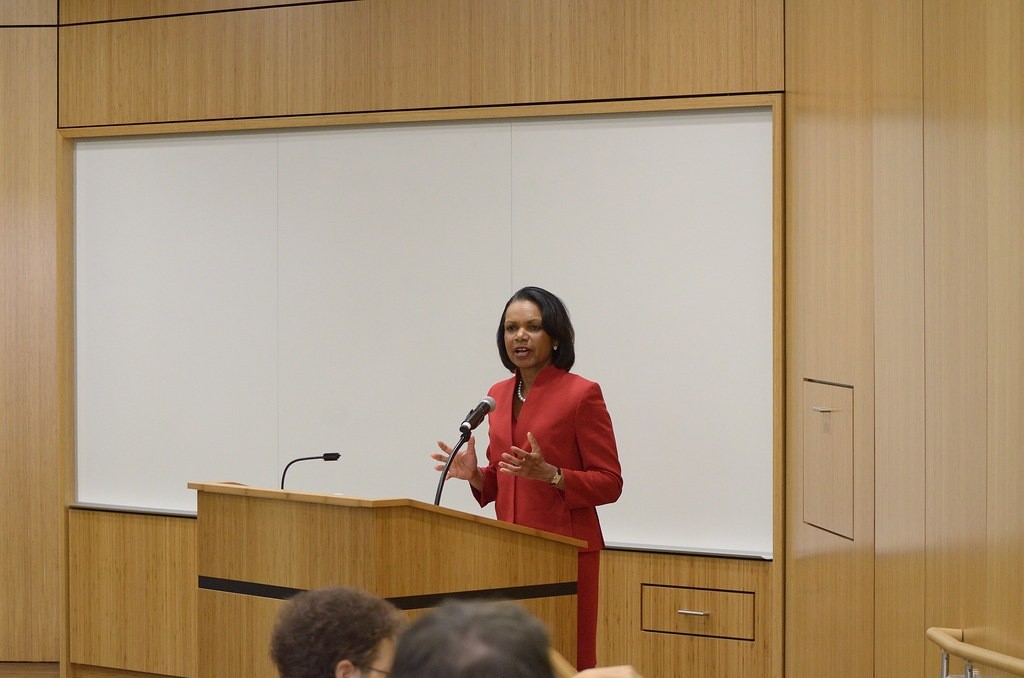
459 396 496 432
281 453 341 490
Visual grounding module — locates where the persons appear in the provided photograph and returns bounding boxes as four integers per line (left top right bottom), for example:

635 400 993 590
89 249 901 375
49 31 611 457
390 602 642 678
431 286 624 670
271 586 405 678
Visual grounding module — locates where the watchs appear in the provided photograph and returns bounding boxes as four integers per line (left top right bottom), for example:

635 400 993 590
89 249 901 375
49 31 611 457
551 468 561 485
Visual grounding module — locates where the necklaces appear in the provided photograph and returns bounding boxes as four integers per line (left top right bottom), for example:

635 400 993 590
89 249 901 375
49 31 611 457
518 380 526 403
